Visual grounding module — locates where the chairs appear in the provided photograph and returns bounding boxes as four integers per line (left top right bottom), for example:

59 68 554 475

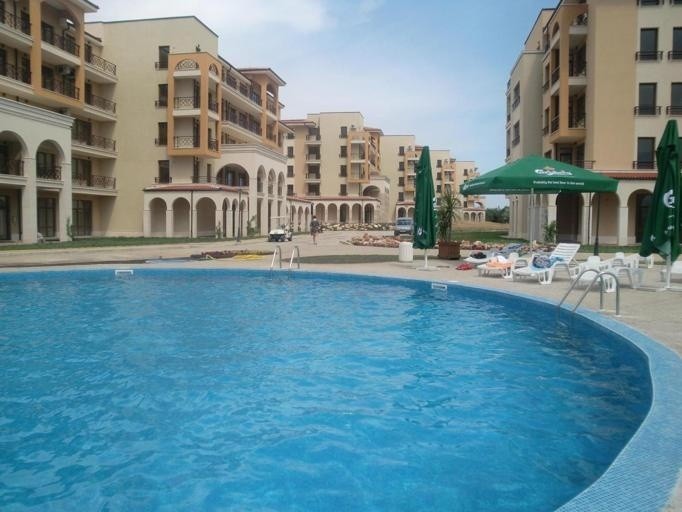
660 259 682 281
465 242 654 293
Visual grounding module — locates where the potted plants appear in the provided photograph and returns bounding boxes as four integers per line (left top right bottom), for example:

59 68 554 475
432 188 463 260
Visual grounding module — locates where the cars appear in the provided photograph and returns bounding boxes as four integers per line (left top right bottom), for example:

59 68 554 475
394 217 414 236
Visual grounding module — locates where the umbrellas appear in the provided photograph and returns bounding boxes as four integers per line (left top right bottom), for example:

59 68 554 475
412 145 438 265
636 118 682 286
458 154 619 253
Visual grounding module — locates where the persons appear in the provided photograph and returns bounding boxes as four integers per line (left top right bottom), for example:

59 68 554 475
309 215 320 245
